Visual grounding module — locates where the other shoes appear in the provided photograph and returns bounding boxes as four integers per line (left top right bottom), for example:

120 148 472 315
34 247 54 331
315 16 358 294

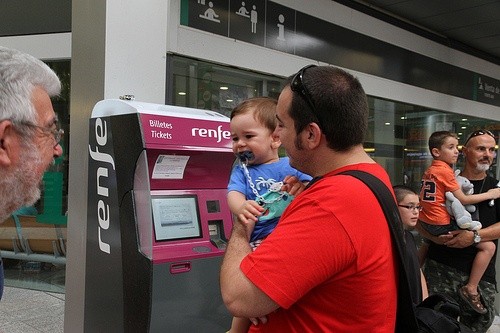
459 286 488 314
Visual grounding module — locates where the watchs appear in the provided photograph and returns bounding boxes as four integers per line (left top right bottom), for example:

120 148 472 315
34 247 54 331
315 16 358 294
473 230 481 244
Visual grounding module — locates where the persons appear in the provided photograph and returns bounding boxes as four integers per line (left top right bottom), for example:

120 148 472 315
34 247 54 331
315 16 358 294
219 64 400 333
415 130 500 333
227 96 313 333
0 46 64 299
393 185 428 309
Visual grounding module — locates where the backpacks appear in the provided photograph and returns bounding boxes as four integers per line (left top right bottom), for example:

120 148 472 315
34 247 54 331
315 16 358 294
302 169 495 333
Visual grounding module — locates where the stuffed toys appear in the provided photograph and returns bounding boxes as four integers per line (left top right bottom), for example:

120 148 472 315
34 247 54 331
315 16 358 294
445 168 482 230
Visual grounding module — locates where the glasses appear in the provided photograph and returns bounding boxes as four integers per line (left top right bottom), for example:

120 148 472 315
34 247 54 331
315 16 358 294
398 204 423 211
291 64 325 131
0 117 64 148
464 131 495 146
